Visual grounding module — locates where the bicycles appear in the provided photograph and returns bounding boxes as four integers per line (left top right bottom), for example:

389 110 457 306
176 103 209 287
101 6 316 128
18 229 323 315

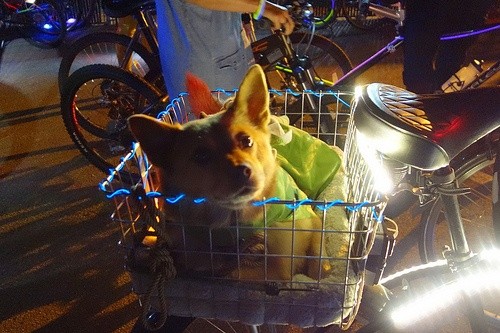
0 0 499 333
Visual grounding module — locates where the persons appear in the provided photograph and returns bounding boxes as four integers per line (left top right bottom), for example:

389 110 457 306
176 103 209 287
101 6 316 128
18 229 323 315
398 0 487 98
153 0 297 101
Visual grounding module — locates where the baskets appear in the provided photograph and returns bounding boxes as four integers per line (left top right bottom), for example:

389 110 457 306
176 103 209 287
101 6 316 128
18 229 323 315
95 88 389 328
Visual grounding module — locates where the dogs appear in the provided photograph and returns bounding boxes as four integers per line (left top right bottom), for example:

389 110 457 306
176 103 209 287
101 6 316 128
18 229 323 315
125 63 332 290
